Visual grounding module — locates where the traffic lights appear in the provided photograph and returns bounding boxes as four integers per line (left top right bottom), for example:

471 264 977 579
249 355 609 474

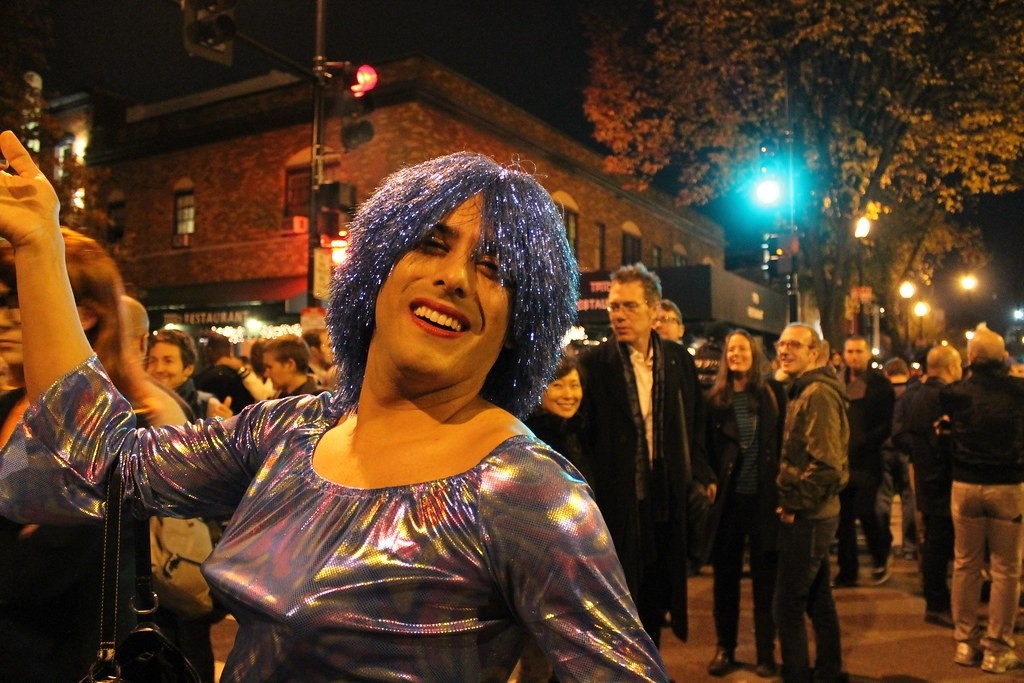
754 137 785 205
316 181 359 248
336 64 378 152
180 0 239 69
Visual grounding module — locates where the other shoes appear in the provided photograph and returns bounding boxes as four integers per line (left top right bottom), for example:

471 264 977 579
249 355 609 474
829 572 855 587
707 642 736 675
903 550 916 560
755 645 776 676
865 552 894 586
924 608 954 627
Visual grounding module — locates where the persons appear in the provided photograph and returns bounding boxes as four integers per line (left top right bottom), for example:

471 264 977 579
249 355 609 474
523 262 1024 683
0 130 668 683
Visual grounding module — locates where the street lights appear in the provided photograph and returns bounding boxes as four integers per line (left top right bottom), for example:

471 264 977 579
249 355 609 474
913 301 929 340
854 216 871 337
897 281 916 366
956 273 981 332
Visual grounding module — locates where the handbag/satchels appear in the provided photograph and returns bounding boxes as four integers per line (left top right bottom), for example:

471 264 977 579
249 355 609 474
69 430 201 683
151 510 212 613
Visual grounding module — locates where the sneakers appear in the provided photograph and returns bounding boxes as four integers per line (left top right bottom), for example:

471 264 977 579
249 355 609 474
981 645 1020 672
953 638 984 667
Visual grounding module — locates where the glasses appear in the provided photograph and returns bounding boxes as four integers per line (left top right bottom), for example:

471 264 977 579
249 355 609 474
774 339 802 352
651 316 681 326
